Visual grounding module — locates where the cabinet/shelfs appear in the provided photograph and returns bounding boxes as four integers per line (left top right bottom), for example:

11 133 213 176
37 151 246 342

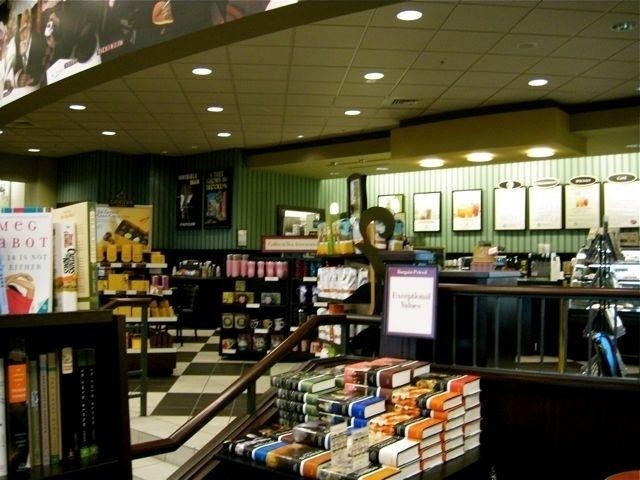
93 248 639 377
0 305 137 480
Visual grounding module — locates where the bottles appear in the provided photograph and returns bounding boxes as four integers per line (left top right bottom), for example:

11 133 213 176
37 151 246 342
226 253 288 281
492 244 563 279
295 260 331 278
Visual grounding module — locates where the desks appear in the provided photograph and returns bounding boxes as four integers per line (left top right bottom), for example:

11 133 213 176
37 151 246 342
210 447 498 479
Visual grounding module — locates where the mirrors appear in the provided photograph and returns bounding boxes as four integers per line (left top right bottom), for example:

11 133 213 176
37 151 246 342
275 203 328 240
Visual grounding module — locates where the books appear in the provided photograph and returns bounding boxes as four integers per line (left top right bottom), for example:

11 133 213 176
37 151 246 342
223 356 484 480
0 201 99 478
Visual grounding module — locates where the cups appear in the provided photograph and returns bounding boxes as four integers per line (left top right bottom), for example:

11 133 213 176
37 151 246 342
445 258 462 266
